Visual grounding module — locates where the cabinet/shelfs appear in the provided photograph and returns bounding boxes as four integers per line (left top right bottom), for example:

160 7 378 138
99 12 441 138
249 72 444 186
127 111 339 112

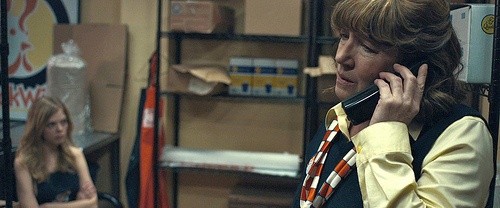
153 0 500 208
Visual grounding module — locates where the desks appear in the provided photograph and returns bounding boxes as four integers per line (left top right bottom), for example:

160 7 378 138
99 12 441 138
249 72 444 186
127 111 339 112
0 123 120 201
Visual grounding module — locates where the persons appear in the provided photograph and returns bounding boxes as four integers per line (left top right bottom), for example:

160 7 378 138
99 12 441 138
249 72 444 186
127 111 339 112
295 0 495 208
13 97 98 208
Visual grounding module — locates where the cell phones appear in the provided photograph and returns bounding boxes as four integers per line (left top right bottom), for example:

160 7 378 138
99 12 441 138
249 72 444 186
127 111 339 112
342 63 434 126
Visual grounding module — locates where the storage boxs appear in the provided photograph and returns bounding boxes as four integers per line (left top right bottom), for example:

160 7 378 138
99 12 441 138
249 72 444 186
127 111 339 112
245 0 305 38
227 183 296 208
170 3 235 34
451 3 495 83
304 55 340 103
167 57 301 98
